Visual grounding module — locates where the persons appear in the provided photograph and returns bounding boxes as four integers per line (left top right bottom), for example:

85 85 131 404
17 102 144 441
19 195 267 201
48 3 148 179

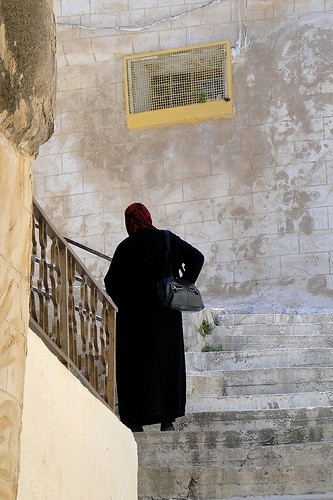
103 203 204 432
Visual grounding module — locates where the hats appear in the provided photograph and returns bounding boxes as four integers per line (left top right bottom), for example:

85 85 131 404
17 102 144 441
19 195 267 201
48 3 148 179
124 202 154 235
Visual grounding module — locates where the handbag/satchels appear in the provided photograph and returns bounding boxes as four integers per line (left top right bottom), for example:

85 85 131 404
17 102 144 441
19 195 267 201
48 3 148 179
157 279 203 312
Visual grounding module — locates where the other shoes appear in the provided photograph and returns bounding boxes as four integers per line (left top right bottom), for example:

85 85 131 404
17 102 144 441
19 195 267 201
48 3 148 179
129 426 143 432
158 423 174 430
